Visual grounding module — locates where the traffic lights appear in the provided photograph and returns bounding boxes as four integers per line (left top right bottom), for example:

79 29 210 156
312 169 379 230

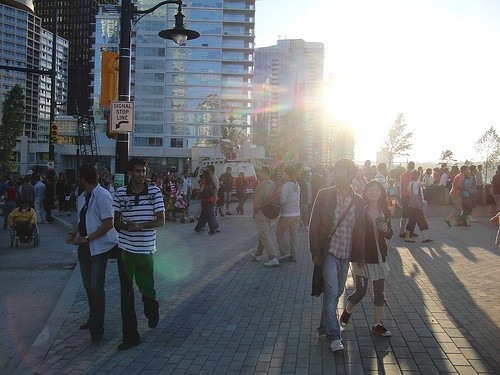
50 124 58 143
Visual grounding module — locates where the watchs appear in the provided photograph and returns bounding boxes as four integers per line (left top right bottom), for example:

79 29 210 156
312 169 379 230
85 236 90 241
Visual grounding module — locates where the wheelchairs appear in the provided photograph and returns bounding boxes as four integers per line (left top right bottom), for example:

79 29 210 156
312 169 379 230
9 222 40 248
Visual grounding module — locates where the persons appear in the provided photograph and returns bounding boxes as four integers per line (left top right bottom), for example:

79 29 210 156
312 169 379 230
8 199 38 245
338 180 392 337
352 160 484 227
398 162 433 243
54 174 71 217
112 158 165 350
307 159 367 352
0 169 53 230
151 169 191 221
193 165 220 234
215 167 247 217
490 165 500 226
252 165 333 267
67 165 118 336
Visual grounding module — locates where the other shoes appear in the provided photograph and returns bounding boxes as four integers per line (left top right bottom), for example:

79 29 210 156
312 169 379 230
236 207 239 214
454 223 467 226
330 340 344 352
264 257 280 267
404 239 416 243
371 320 393 337
148 307 159 328
118 338 141 350
225 212 232 215
444 218 451 227
340 306 352 327
467 215 479 223
318 333 343 343
219 212 225 217
422 239 433 242
79 320 89 329
193 226 221 236
399 232 418 238
279 253 297 263
250 252 262 262
91 331 104 341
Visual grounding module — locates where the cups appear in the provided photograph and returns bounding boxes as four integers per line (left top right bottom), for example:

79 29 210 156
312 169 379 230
377 216 387 232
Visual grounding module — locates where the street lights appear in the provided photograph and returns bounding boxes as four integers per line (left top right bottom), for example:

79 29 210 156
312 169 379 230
113 0 201 198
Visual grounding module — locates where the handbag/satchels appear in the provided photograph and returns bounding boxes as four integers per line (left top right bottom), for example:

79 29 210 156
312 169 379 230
311 263 324 297
262 205 280 219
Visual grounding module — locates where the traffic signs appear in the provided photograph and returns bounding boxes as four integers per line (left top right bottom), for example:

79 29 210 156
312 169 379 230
110 101 135 134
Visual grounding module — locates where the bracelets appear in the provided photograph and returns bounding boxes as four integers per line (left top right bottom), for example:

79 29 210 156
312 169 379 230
69 232 76 237
